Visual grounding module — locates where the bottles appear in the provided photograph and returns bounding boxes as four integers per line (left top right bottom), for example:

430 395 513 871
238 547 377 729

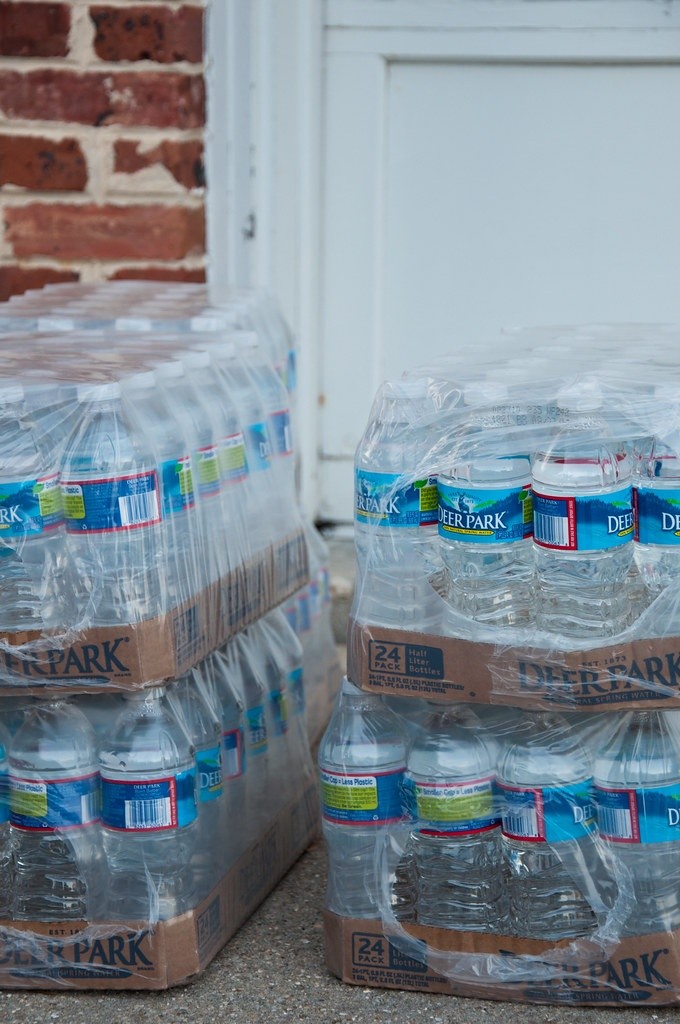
0 272 343 999
318 316 679 1008
